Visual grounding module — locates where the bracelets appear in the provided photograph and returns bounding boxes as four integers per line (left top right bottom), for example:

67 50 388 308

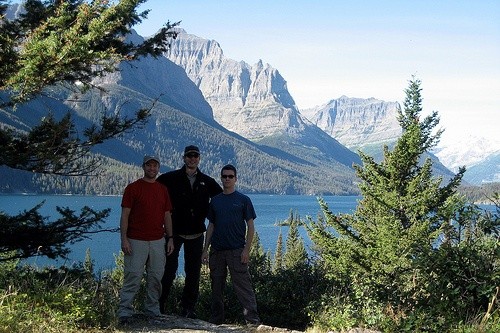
167 236 173 239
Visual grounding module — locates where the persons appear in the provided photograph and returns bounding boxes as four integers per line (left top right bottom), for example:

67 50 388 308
201 164 261 328
155 145 224 319
118 155 173 325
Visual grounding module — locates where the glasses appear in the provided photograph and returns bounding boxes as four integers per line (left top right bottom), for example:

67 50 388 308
221 175 235 179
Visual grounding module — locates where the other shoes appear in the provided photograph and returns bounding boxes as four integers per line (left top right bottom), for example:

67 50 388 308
161 308 170 315
245 313 260 324
185 310 196 318
118 316 129 324
147 315 168 325
212 319 225 325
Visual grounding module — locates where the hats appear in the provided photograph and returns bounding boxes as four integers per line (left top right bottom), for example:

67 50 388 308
143 155 160 165
183 145 200 155
